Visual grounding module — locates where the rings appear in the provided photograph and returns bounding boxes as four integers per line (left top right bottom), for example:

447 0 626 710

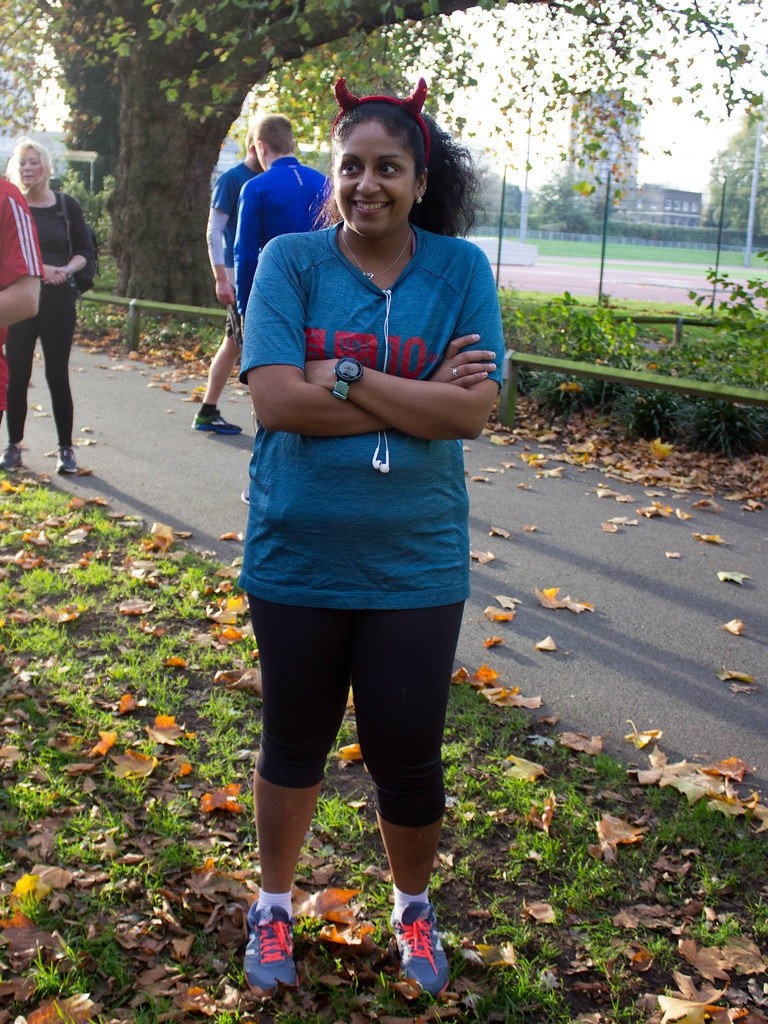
453 368 458 377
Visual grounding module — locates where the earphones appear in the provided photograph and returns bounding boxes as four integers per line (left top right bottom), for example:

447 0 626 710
380 450 390 473
372 448 382 470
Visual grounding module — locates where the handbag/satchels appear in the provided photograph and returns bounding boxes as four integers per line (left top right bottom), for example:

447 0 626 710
58 192 99 294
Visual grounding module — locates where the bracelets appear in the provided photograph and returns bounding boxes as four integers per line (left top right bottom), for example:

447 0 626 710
66 265 71 278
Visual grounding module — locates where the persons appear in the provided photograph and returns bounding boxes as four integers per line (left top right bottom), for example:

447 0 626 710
234 113 329 504
0 140 94 473
0 176 43 424
240 78 504 996
192 122 264 435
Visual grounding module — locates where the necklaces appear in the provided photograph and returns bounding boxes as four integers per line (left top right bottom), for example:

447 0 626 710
342 227 411 280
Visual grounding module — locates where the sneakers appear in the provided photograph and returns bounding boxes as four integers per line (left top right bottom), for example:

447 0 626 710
191 411 243 433
389 899 448 996
242 897 298 995
54 445 77 473
0 442 23 471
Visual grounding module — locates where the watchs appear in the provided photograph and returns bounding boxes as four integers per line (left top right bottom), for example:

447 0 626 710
332 356 364 400
